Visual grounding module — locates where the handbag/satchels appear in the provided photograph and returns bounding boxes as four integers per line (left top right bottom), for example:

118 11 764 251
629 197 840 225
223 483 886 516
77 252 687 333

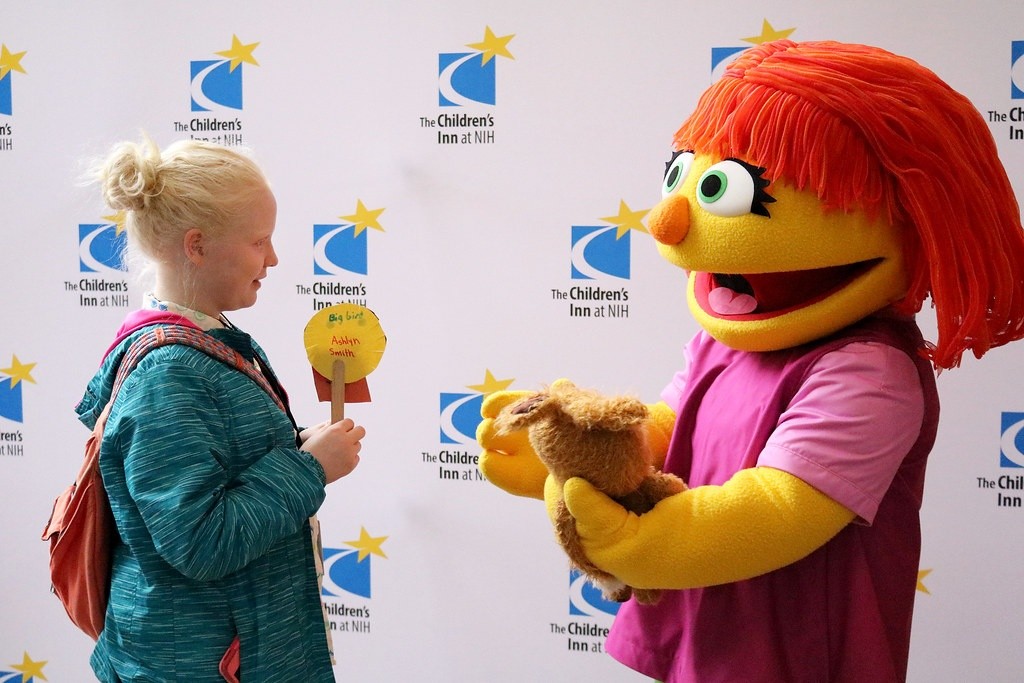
49 402 119 643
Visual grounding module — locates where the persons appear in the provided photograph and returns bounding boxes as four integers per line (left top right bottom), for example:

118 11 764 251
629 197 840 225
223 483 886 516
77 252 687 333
72 137 367 683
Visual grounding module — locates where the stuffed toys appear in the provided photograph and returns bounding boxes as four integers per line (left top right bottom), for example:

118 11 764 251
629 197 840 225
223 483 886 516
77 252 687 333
494 375 689 608
472 42 1024 683
